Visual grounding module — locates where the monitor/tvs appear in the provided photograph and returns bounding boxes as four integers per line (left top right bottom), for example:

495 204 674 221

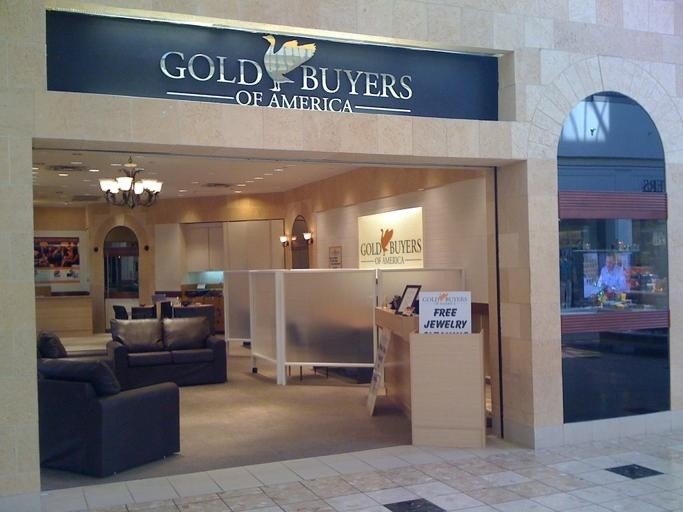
572 250 641 306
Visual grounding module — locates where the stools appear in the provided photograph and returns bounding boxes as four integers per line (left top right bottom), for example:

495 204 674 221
279 235 289 248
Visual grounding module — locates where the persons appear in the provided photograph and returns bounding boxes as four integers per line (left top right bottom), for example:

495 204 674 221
597 252 627 294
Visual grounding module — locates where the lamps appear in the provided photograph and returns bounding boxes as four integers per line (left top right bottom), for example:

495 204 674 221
98 165 164 208
302 232 313 245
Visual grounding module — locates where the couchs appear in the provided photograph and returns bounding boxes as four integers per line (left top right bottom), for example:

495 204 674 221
36 294 227 477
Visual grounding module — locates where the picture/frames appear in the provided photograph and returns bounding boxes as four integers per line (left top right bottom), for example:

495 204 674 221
395 285 422 314
